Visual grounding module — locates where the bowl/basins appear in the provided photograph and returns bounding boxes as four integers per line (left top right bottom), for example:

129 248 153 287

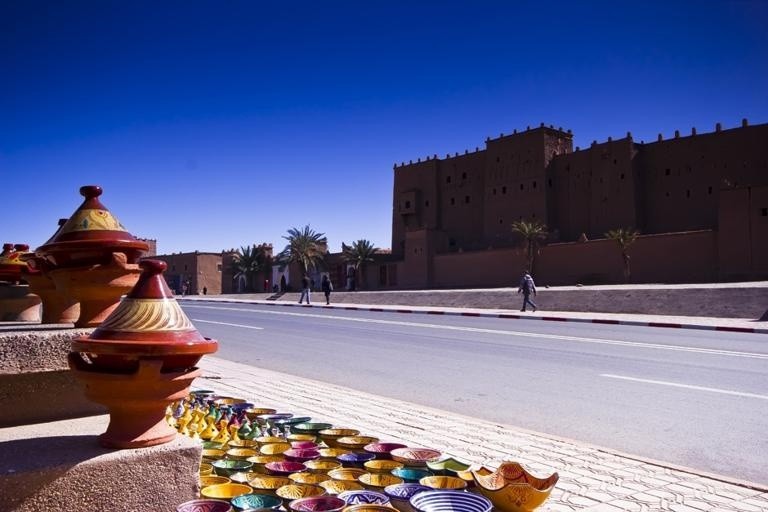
291 418 362 434
176 435 560 511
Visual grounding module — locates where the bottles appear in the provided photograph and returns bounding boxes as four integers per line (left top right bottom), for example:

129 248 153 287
165 390 290 443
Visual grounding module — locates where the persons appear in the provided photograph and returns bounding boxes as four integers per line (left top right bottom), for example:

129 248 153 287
298 272 310 304
321 275 331 305
517 270 537 313
